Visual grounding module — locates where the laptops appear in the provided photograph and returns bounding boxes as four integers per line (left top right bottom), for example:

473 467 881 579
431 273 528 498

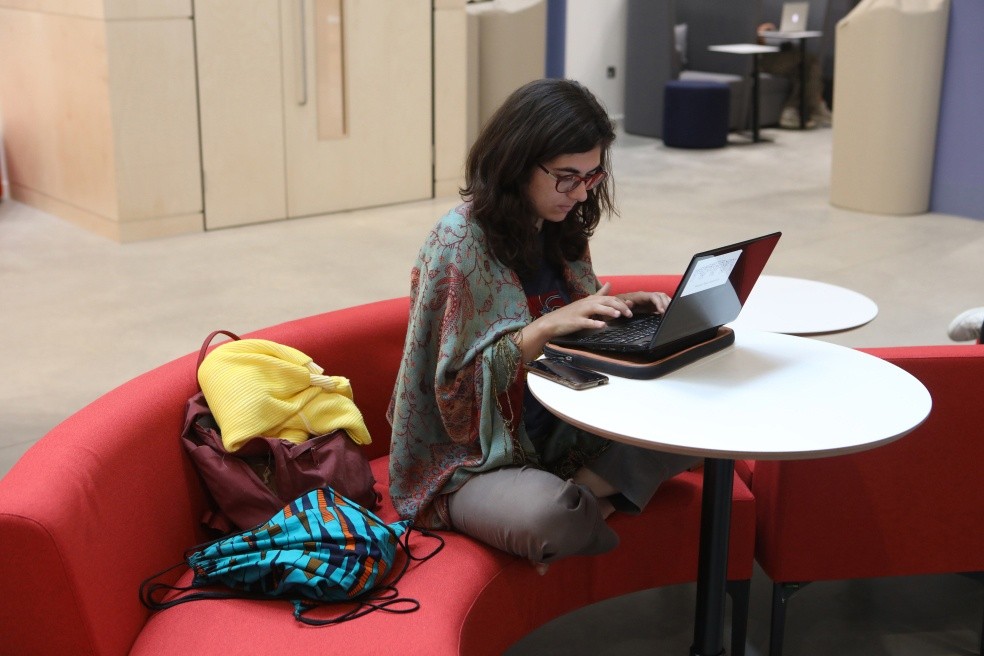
778 2 809 32
549 232 784 352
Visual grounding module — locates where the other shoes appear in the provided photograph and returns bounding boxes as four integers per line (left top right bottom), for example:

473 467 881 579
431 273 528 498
947 306 984 341
809 103 832 124
779 106 816 128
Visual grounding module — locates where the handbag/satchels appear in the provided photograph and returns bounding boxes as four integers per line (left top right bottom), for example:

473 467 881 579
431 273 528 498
182 330 384 538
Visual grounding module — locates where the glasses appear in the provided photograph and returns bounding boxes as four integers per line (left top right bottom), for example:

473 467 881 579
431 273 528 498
536 162 608 193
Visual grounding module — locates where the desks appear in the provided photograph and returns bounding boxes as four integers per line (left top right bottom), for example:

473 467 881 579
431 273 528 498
500 272 932 655
708 41 779 141
755 29 823 131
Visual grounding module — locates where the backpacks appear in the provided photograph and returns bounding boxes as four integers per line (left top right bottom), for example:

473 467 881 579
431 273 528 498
139 483 445 625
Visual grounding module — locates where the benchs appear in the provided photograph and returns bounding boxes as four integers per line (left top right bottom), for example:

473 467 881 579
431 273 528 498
735 341 984 656
0 255 782 656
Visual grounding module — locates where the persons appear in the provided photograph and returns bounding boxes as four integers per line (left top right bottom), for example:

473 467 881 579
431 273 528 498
386 76 707 564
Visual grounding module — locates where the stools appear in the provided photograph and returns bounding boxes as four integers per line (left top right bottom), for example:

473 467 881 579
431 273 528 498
659 67 785 151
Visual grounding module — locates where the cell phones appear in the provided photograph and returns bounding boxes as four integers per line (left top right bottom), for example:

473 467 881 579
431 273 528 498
521 358 609 391
